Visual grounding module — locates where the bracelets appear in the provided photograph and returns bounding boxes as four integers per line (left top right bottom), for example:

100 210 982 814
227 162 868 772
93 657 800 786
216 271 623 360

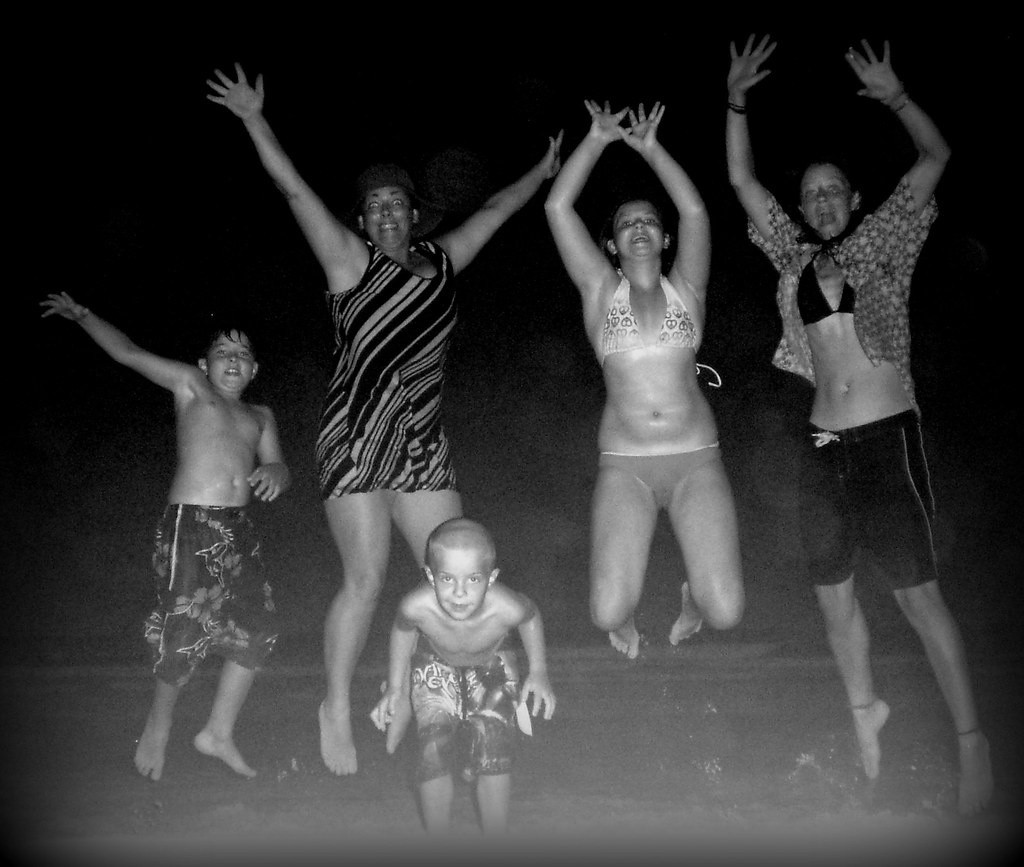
889 96 913 118
724 100 750 118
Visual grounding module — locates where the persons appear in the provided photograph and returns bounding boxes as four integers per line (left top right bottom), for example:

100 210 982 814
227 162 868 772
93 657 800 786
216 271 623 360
544 98 748 658
719 27 1011 821
39 288 293 781
384 515 554 836
198 58 565 775
